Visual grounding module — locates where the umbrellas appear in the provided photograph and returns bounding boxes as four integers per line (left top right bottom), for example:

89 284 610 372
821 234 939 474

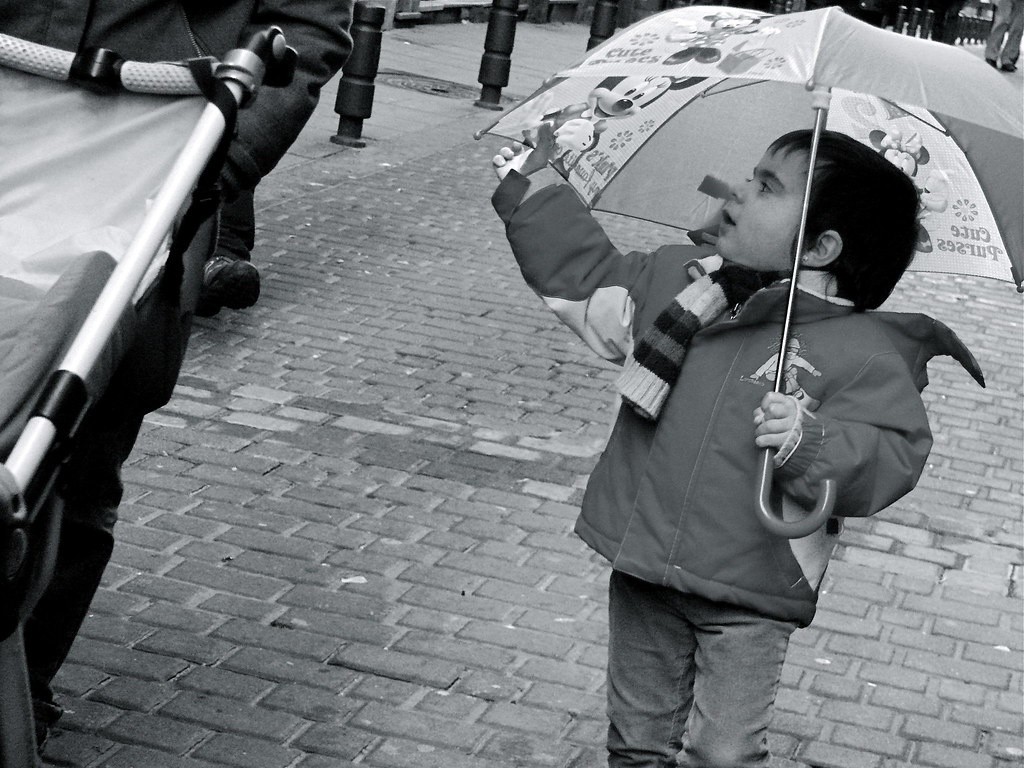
475 5 1024 296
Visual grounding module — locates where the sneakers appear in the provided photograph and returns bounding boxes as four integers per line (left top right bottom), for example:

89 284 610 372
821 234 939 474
195 256 259 317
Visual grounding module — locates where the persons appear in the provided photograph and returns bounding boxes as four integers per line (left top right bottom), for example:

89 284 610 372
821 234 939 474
985 0 1024 72
0 0 360 756
489 120 985 768
